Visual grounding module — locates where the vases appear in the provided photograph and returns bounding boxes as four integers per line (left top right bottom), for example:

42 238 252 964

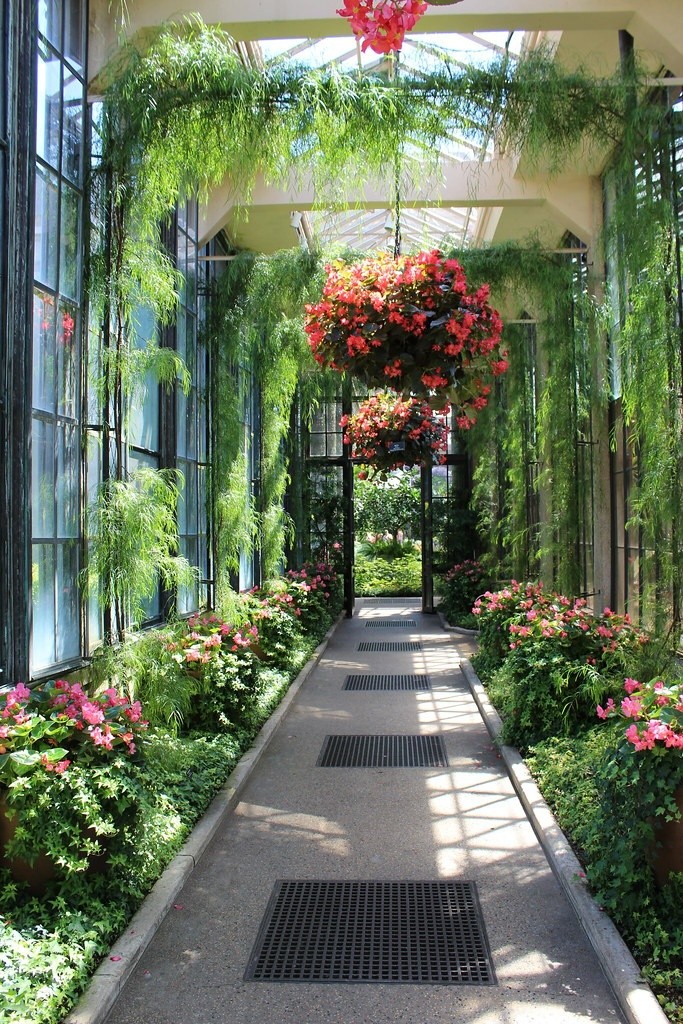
248 641 267 661
185 669 202 681
385 440 407 453
643 775 683 892
0 791 58 892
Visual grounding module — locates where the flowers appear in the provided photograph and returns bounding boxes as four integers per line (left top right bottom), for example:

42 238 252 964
337 0 429 56
302 249 508 428
336 388 449 483
0 563 342 774
443 559 683 754
